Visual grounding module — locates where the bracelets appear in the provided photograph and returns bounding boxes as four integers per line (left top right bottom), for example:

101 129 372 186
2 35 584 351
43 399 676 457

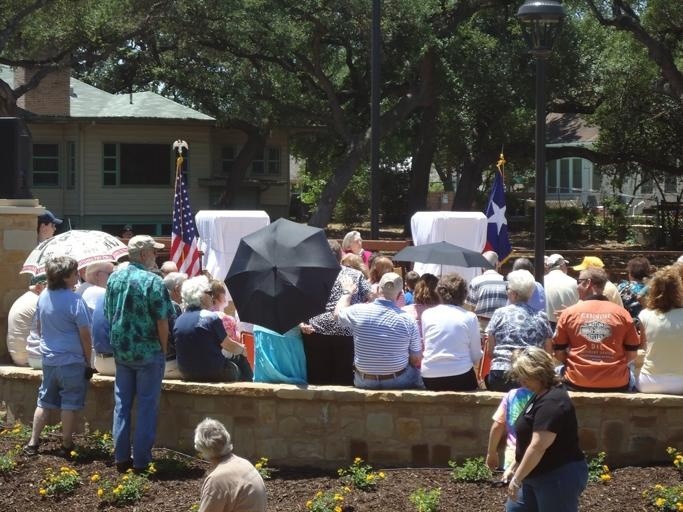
510 476 524 491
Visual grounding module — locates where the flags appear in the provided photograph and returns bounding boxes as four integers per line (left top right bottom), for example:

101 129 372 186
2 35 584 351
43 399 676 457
168 156 199 277
482 163 513 264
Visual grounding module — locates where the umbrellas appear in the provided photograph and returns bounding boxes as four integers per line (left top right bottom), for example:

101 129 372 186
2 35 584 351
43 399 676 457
223 217 341 337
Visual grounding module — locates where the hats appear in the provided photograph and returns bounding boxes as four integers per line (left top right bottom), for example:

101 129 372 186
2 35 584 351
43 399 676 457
126 233 166 251
377 271 405 295
35 209 64 225
572 255 607 273
547 254 569 267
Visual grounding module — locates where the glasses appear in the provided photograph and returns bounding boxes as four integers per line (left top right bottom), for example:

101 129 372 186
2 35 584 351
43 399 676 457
576 278 590 284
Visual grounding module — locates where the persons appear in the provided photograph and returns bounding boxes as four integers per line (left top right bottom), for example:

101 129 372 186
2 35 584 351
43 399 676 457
34 209 63 243
20 255 97 460
481 347 533 487
192 416 270 512
494 344 588 510
1 230 683 396
120 226 134 238
101 233 176 475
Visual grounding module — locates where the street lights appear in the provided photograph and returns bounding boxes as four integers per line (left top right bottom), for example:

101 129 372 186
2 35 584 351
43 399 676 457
516 1 566 280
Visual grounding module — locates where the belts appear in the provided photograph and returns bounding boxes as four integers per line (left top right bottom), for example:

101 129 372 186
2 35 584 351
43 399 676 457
352 365 407 382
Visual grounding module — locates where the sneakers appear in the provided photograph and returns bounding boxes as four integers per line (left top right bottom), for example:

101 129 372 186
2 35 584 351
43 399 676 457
133 466 152 474
59 443 76 456
20 444 42 456
112 458 133 473
